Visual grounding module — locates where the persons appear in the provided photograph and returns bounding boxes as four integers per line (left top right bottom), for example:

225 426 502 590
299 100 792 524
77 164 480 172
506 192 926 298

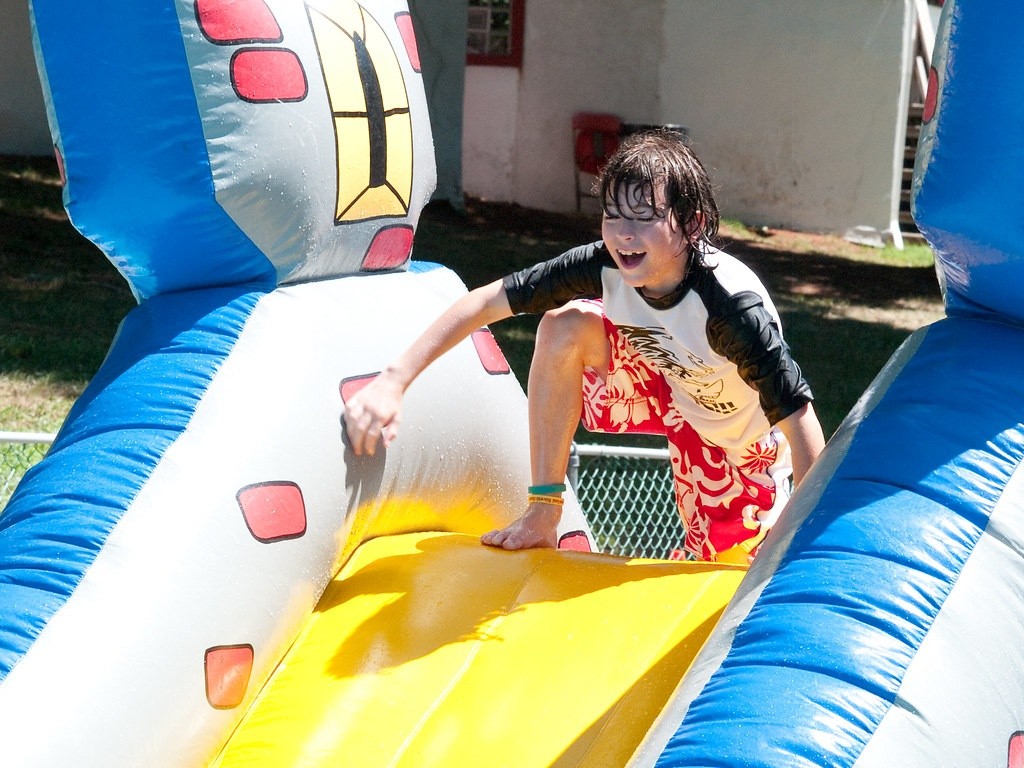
344 136 826 564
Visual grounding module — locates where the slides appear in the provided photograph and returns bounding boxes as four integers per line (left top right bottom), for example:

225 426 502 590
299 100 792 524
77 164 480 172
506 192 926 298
211 526 755 768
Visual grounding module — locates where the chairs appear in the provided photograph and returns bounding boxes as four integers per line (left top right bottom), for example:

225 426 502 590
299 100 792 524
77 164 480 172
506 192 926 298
571 111 629 208
